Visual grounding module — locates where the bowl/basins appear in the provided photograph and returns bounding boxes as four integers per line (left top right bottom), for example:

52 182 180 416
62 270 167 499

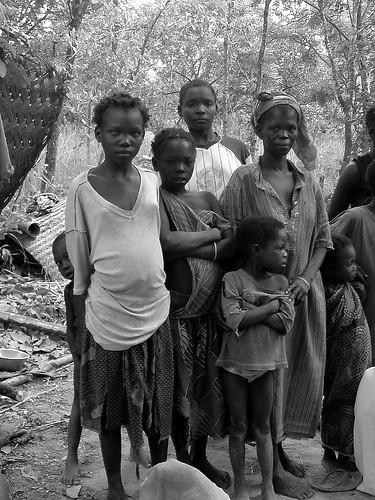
0 349 30 371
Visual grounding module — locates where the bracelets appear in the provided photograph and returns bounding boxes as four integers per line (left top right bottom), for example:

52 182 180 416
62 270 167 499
296 277 311 289
213 241 217 262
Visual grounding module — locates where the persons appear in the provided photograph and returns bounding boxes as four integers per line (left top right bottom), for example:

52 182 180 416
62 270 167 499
214 215 296 500
151 127 236 489
327 105 375 222
217 90 336 478
65 92 175 500
178 79 253 201
330 160 375 366
319 234 373 472
52 231 154 485
